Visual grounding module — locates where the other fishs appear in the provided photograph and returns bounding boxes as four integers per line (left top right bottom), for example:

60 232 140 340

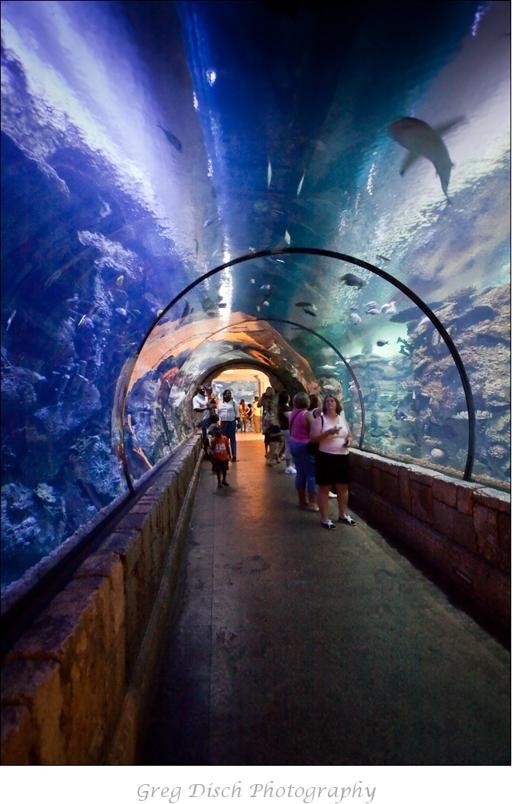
113 217 428 459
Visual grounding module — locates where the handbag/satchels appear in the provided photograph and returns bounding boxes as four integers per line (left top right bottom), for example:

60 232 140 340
305 442 315 455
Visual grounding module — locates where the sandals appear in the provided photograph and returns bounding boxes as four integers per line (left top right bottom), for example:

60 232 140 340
339 514 356 525
320 520 336 529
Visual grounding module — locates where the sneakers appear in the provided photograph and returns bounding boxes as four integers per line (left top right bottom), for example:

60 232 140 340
328 491 338 498
285 464 297 474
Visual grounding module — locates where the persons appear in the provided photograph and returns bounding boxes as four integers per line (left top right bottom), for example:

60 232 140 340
308 394 359 531
190 383 338 512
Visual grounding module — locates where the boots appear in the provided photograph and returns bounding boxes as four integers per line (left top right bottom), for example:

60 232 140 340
298 490 319 511
